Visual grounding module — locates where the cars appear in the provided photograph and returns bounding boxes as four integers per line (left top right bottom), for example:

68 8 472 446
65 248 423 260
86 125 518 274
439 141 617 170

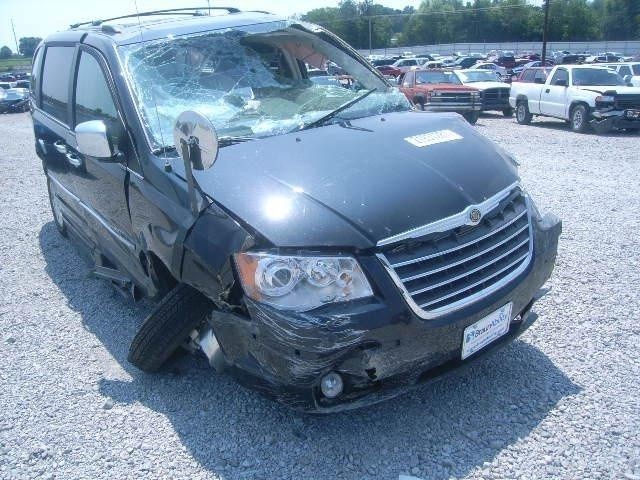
452 69 514 118
0 70 33 114
363 51 640 84
397 68 481 124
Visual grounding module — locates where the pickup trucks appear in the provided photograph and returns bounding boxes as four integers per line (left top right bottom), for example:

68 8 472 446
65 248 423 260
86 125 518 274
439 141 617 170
509 64 640 132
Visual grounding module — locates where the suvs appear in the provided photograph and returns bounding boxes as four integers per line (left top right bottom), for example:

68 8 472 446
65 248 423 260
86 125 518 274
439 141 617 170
29 5 563 415
